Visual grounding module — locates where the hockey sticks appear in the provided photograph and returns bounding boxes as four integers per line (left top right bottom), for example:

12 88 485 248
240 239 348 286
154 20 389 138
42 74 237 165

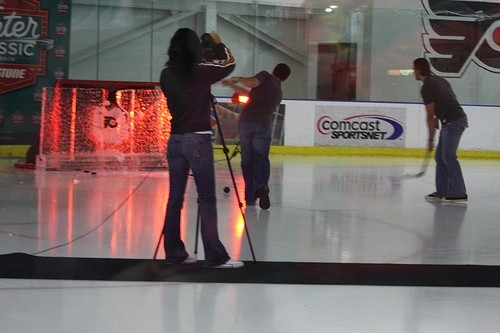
404 128 436 179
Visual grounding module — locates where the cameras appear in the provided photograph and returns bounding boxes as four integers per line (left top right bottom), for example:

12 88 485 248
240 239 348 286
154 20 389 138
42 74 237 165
200 33 216 60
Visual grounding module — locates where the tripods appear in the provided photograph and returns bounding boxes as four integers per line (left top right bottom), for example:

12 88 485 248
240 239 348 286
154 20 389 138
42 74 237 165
152 95 257 265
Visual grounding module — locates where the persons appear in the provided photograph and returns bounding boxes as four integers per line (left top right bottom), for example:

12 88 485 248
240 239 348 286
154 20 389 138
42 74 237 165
220 64 291 209
159 27 245 270
413 57 470 203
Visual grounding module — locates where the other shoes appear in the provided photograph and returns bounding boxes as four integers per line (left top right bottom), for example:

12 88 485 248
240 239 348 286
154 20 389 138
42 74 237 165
256 184 271 210
246 200 255 206
427 194 445 200
445 194 467 203
204 258 244 269
180 254 197 263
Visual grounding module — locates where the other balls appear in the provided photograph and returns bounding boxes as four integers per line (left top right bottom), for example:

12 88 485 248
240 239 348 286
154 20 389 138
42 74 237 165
84 170 89 173
224 187 229 193
91 172 96 174
